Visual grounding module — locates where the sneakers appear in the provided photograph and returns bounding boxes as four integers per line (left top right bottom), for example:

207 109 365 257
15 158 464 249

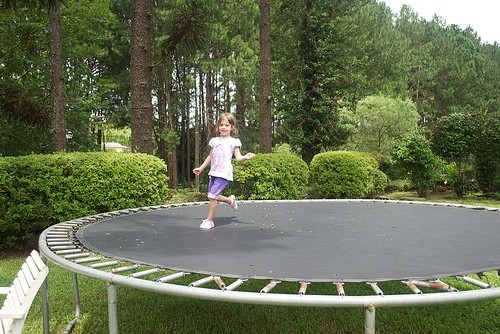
200 219 214 229
229 195 238 210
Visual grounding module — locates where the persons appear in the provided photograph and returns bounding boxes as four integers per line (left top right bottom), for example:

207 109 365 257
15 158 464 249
193 112 256 228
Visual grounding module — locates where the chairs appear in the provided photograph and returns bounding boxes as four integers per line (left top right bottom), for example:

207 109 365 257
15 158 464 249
0 250 49 334
